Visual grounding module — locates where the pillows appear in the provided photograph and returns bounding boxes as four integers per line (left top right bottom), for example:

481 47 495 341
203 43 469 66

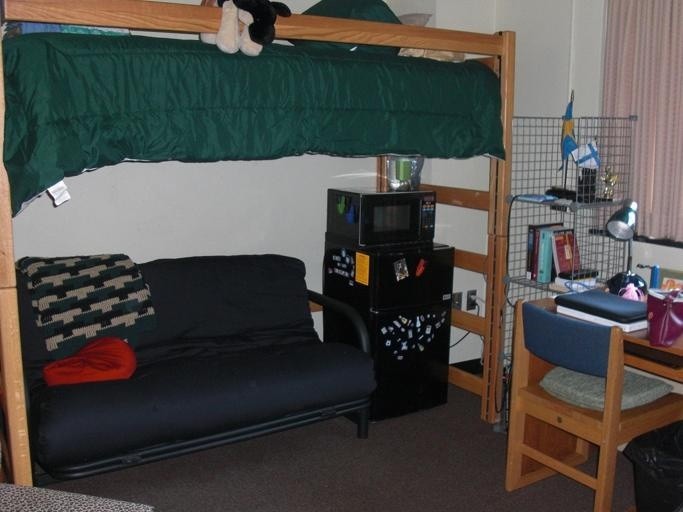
43 337 138 387
397 14 431 53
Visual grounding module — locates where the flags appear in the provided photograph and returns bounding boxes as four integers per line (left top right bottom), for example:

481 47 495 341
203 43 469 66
571 141 602 170
557 102 580 161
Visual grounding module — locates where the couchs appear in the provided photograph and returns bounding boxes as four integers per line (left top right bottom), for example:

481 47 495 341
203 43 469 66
15 253 378 485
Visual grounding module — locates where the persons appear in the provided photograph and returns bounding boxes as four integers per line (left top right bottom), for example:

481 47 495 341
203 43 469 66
596 164 620 200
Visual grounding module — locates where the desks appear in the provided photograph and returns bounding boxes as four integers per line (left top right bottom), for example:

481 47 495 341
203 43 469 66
527 286 682 397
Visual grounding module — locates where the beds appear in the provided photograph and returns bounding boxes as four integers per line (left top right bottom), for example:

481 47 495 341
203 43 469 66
0 0 516 486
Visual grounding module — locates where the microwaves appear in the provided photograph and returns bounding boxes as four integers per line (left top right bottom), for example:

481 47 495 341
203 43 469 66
326 186 436 250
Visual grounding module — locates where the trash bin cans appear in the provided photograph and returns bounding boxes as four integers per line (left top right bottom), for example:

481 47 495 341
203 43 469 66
622 420 683 512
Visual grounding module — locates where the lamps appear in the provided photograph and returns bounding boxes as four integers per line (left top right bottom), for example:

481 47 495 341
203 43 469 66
606 199 647 296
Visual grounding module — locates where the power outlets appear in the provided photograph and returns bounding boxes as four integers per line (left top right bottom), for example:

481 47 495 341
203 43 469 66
452 292 462 310
467 291 477 311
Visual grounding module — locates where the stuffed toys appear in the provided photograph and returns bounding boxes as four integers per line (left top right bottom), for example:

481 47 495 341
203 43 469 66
196 0 292 58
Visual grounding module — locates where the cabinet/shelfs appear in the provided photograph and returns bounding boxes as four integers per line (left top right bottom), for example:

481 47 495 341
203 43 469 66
509 195 631 297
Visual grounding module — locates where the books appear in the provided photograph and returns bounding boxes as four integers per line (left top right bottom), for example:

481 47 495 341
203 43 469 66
553 289 648 325
524 222 606 296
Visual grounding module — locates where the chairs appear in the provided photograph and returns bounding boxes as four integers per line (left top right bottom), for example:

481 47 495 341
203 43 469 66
504 304 682 512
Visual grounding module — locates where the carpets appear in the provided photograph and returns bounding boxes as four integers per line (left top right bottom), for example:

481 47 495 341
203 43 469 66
0 483 157 512
